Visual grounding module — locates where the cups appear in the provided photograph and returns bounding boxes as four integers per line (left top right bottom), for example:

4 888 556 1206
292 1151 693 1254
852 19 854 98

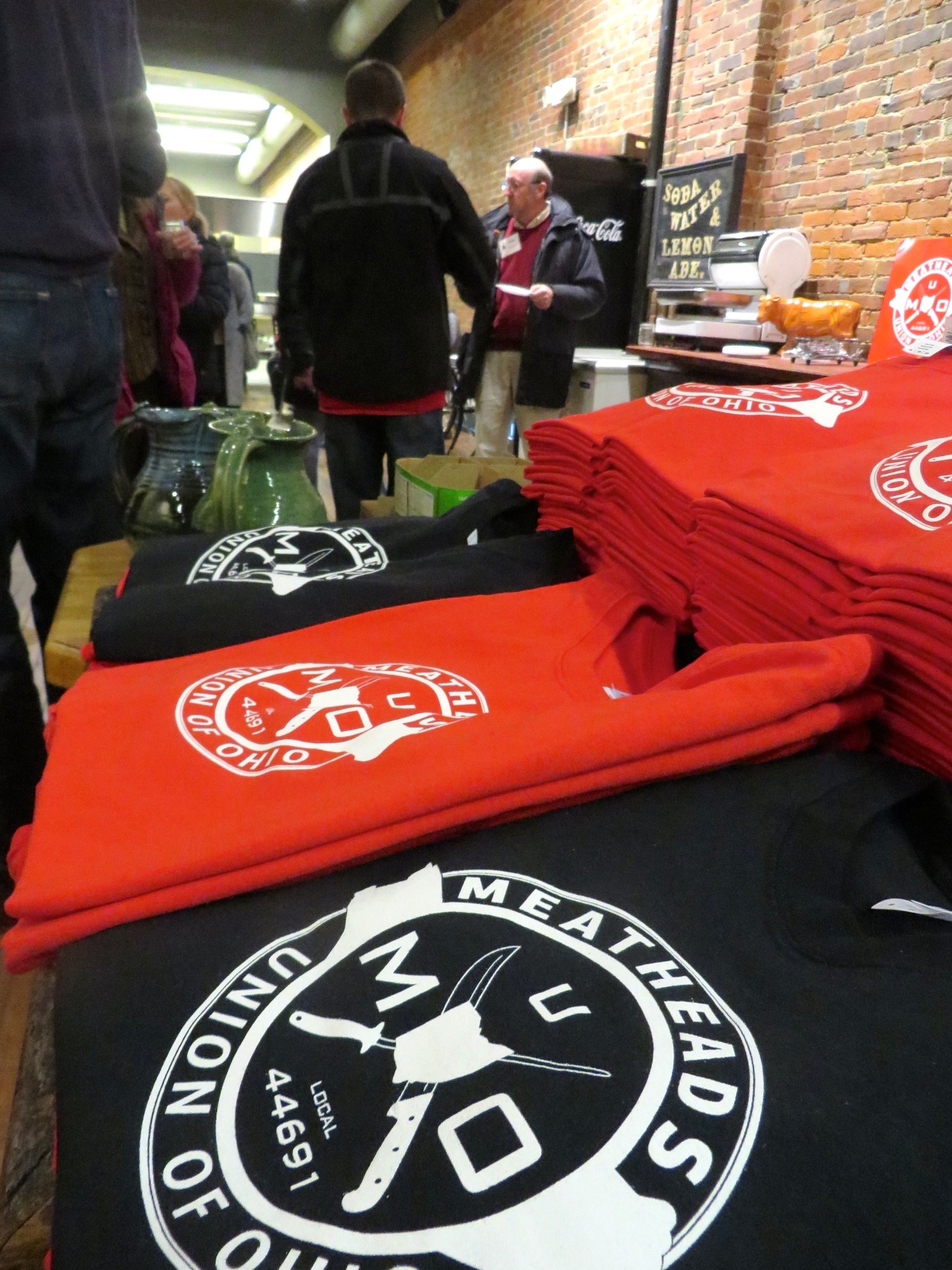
639 323 657 344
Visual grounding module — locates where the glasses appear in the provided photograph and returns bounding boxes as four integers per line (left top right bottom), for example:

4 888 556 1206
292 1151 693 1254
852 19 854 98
502 180 532 196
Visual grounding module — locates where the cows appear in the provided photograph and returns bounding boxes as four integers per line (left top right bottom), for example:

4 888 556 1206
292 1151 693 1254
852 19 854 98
757 293 862 357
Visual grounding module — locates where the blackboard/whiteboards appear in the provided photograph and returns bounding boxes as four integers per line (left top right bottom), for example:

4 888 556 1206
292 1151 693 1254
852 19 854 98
648 153 747 291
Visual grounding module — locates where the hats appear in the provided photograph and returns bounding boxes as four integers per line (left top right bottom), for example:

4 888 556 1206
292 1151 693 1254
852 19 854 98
218 232 235 249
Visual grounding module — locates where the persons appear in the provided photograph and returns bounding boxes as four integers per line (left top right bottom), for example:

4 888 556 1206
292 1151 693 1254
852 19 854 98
276 57 610 520
0 0 258 950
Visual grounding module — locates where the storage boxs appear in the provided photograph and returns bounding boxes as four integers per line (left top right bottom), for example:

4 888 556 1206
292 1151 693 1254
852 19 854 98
395 455 533 519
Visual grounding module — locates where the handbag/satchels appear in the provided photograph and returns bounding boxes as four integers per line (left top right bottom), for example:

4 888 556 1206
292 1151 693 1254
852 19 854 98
245 330 258 371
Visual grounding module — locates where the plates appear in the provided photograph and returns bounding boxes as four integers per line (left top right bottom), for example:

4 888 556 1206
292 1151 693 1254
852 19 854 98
496 283 530 296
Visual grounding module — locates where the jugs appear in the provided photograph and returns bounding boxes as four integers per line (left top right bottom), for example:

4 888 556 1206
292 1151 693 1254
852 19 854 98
107 407 234 556
189 413 330 534
